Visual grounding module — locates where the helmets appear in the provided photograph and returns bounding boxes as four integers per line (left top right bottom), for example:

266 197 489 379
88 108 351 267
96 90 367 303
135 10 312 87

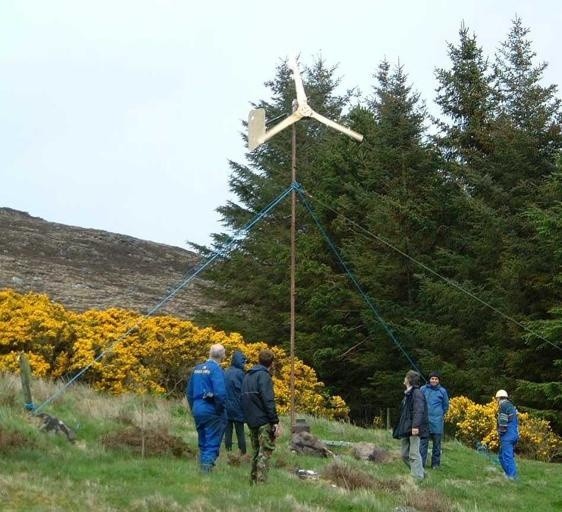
496 390 508 398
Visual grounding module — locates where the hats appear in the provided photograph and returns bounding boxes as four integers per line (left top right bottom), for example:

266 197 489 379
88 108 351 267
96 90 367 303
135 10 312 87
428 372 440 381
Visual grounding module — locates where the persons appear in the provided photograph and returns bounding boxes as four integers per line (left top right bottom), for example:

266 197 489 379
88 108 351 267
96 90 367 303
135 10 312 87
420 373 449 470
393 370 429 483
186 344 228 474
495 389 520 481
241 351 280 488
224 351 247 455
